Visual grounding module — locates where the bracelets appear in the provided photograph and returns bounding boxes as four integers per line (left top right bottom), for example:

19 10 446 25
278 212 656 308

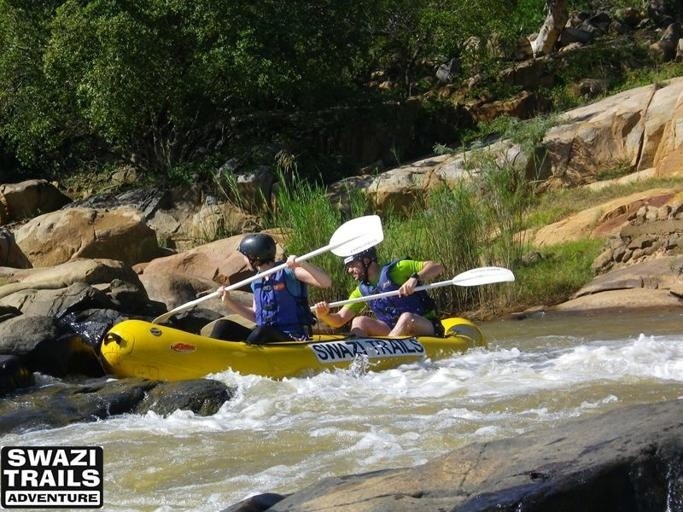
411 270 424 287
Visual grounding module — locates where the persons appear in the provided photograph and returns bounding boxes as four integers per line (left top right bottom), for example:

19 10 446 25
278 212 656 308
209 231 332 346
312 242 446 338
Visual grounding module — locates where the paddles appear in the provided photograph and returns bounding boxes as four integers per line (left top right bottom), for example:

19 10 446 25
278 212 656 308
152 215 384 323
310 267 515 312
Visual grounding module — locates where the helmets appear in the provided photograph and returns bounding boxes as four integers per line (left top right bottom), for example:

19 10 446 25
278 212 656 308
342 246 376 264
237 233 275 262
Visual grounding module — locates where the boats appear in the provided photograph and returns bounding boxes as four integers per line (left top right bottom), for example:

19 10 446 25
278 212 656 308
100 315 486 383
